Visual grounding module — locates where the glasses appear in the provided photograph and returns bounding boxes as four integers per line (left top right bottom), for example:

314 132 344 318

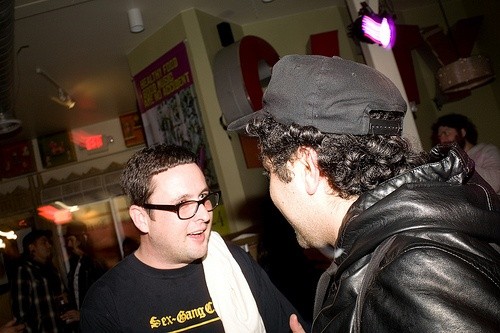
142 189 222 220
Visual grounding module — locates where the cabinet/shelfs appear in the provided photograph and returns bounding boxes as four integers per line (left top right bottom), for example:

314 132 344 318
0 145 144 288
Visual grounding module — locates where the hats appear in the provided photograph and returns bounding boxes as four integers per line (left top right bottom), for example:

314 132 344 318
63 221 91 238
22 229 53 251
226 54 409 137
430 113 479 135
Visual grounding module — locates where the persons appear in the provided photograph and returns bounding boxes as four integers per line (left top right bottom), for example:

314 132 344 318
430 112 500 194
80 145 310 333
228 54 500 333
0 222 139 333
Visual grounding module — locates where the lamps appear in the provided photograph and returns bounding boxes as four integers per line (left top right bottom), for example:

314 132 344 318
128 8 144 33
435 0 496 94
59 89 75 108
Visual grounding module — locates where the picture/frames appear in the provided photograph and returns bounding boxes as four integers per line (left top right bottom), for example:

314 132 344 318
38 130 77 169
